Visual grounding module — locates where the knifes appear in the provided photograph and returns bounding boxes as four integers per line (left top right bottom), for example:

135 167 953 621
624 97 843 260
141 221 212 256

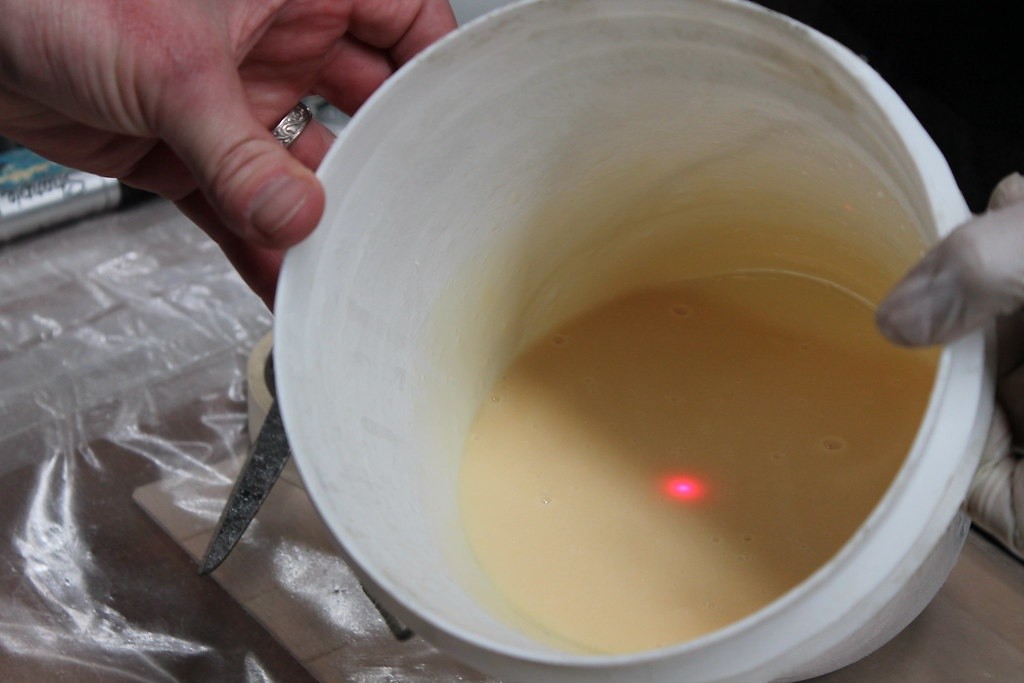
197 349 291 576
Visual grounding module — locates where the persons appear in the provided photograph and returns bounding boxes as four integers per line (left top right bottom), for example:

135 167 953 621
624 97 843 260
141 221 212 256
0 0 458 313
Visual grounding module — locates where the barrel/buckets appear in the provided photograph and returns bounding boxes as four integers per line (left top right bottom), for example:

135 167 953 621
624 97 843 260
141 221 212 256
271 0 996 683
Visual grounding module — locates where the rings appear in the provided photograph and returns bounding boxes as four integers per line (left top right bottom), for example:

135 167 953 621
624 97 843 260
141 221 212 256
273 104 311 147
